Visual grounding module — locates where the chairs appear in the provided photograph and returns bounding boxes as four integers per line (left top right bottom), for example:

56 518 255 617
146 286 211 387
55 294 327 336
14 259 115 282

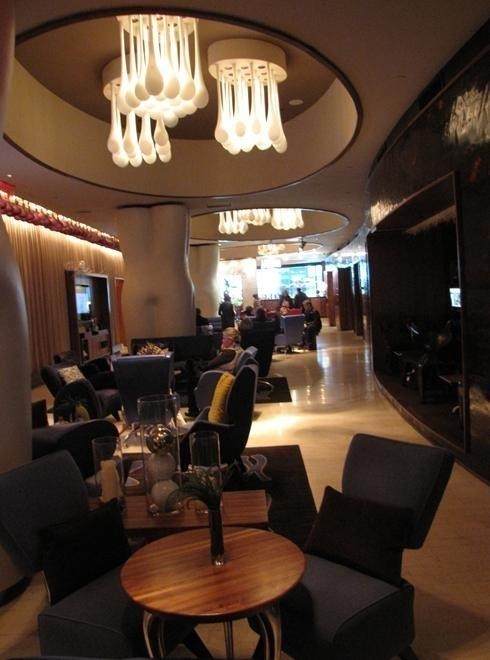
1 450 215 659
41 362 122 422
112 352 174 425
233 346 259 375
274 314 305 354
236 321 280 395
247 434 455 659
180 365 259 472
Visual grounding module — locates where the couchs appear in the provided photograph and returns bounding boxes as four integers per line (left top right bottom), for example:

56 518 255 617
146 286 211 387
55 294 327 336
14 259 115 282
122 334 217 395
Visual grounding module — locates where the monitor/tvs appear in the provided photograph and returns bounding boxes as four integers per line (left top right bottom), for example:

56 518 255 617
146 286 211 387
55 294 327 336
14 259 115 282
449 287 461 307
75 285 92 314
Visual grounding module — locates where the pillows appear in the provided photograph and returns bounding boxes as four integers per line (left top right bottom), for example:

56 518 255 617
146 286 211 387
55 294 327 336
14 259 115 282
302 485 414 588
208 371 235 424
57 365 86 385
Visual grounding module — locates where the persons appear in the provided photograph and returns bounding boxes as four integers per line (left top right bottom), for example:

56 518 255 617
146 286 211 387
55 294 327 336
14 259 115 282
240 305 254 330
195 308 209 326
279 288 322 351
184 327 244 419
252 307 272 329
252 294 262 316
218 294 236 330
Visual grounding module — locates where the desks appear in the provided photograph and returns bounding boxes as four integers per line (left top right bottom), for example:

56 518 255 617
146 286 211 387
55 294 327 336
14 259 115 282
120 526 306 660
122 490 269 529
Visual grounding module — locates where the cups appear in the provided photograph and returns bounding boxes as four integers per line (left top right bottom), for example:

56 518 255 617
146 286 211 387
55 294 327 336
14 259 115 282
139 393 186 520
207 508 223 568
191 430 223 519
91 435 127 517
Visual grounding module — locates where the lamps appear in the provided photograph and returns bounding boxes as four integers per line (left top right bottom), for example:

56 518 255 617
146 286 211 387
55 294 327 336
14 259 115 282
207 38 288 155
257 244 285 255
214 209 305 235
102 52 172 168
116 14 209 129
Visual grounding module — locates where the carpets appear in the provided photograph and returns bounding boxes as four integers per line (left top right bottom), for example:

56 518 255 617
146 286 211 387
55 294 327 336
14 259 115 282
123 446 317 552
255 377 292 403
